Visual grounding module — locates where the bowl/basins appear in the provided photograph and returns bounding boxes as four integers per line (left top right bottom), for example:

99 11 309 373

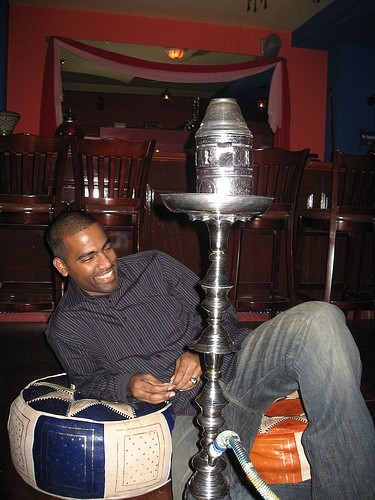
0 111 21 135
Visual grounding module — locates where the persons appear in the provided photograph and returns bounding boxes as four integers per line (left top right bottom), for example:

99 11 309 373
43 210 375 500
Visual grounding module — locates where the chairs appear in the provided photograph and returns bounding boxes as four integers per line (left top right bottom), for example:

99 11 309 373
70 136 157 253
0 133 69 311
297 148 375 310
231 148 310 312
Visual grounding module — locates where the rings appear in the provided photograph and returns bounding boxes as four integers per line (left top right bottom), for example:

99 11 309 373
192 378 198 385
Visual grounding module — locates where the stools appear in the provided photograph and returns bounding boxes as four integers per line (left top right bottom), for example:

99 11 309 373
7 373 175 500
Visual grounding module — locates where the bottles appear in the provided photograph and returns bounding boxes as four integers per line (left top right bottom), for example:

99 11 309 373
194 98 253 196
